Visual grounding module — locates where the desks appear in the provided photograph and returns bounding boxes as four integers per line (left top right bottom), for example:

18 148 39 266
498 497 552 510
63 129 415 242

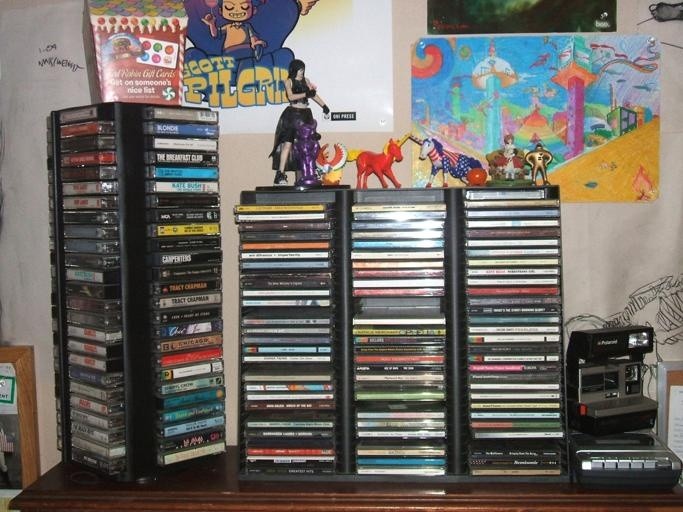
9 461 683 512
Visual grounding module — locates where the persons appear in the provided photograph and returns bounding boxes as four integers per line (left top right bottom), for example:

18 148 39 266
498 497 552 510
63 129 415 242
522 143 552 188
485 134 534 180
264 58 337 190
